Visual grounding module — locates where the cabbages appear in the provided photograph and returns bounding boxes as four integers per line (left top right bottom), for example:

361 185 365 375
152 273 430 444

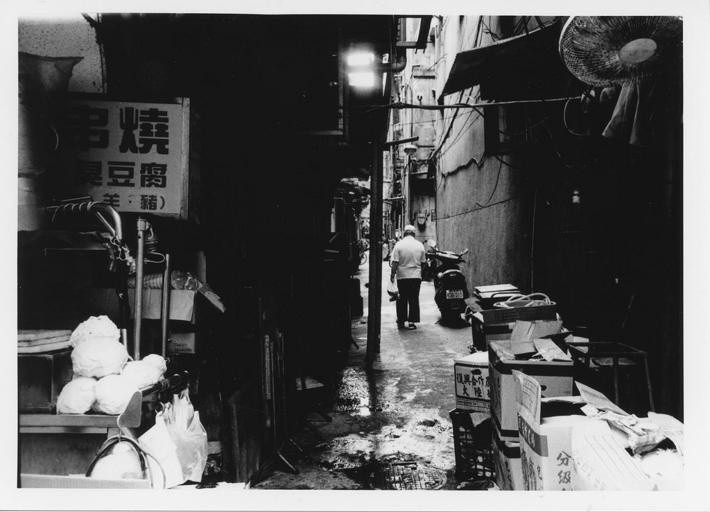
57 316 167 416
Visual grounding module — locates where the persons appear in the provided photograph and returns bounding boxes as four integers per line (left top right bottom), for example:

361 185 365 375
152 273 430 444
389 224 427 330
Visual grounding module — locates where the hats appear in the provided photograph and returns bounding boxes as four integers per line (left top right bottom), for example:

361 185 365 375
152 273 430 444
404 224 417 234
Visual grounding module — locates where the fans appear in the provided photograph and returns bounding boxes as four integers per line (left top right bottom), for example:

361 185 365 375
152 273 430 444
558 16 683 86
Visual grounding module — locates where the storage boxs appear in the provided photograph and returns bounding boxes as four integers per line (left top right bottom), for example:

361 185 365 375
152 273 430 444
452 282 576 491
89 287 228 353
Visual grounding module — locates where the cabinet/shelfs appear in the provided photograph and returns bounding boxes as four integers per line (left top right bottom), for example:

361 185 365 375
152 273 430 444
568 339 655 413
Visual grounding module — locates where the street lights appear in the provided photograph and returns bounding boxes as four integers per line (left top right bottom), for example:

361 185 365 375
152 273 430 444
404 145 418 223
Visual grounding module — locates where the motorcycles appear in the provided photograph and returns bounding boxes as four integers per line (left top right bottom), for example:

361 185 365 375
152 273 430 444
426 245 469 328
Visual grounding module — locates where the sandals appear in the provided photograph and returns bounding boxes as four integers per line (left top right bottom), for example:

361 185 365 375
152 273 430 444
397 322 418 331
